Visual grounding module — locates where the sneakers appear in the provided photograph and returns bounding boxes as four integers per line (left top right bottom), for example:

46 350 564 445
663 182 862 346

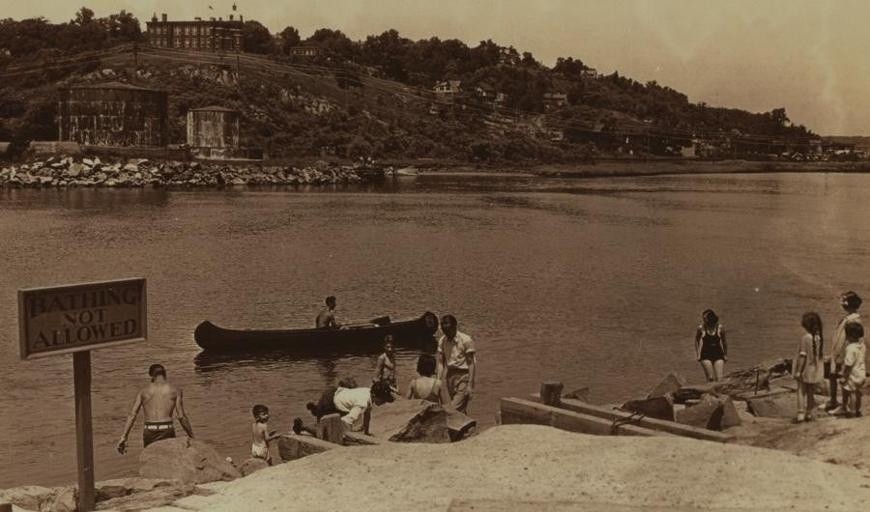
791 411 815 423
817 398 863 419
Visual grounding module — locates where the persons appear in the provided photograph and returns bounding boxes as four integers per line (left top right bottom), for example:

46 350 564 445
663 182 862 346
116 365 194 455
316 296 341 329
790 311 825 423
372 335 398 390
838 323 868 417
828 291 862 410
292 381 393 436
249 406 280 462
407 354 447 406
694 310 727 384
435 314 477 416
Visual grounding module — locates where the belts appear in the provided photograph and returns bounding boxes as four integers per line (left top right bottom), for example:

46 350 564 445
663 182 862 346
143 422 174 430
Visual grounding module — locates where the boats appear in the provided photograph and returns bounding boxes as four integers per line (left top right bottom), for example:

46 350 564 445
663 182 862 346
193 308 438 355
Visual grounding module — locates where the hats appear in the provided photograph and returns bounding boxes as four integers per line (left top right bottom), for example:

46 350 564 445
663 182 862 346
369 380 396 403
